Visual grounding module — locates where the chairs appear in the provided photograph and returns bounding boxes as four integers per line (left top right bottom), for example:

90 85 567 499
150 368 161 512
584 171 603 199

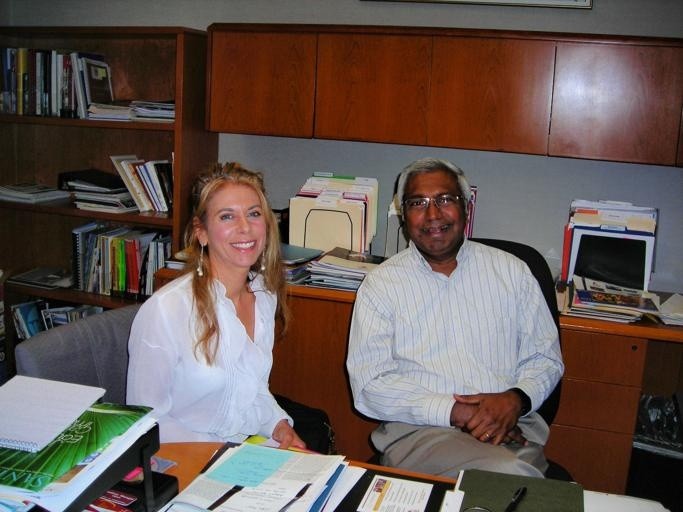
468 237 575 481
14 302 337 453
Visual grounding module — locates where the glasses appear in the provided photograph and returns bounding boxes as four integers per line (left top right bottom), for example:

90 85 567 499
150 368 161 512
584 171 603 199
401 194 464 211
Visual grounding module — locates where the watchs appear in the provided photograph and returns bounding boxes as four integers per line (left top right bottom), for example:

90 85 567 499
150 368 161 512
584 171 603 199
508 387 532 417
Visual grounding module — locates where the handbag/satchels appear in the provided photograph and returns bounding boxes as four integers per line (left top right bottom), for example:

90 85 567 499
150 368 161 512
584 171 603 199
636 393 682 443
273 394 335 455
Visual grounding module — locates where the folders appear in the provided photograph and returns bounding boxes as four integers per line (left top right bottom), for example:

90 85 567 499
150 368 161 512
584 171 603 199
57 168 129 193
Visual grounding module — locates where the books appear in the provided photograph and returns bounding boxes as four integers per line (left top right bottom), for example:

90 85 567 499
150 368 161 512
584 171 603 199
276 171 383 292
552 197 682 329
0 155 175 214
11 300 103 341
0 46 176 123
158 433 466 512
0 374 159 512
7 222 172 299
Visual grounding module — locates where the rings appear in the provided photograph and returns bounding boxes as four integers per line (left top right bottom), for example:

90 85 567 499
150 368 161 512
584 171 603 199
484 432 490 440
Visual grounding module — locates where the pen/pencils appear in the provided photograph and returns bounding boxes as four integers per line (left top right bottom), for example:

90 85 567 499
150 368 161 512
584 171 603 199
503 486 527 512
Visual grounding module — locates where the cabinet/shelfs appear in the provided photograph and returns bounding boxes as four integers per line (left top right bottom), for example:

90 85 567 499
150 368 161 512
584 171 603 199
0 26 219 365
28 423 179 512
204 23 683 168
153 268 683 496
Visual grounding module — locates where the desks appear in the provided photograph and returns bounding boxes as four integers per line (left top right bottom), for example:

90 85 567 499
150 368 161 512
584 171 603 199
159 442 456 512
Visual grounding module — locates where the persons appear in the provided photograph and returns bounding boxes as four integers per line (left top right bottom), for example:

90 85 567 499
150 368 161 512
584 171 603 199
124 160 308 452
346 157 565 480
